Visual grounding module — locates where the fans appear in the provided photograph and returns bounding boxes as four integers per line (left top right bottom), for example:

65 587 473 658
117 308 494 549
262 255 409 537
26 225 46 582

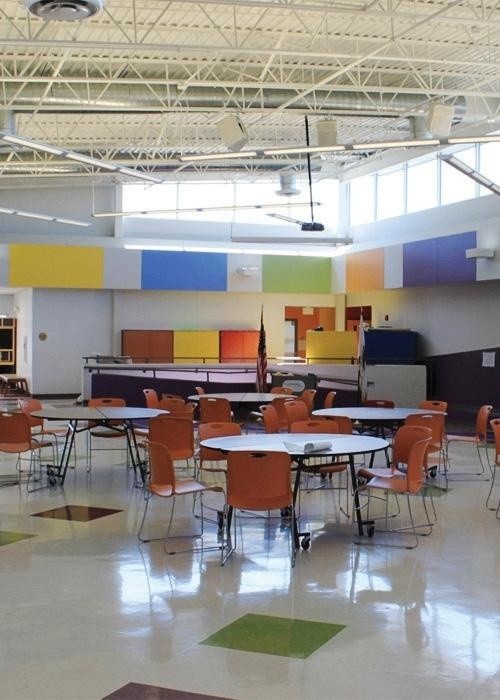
20 0 103 24
266 116 348 238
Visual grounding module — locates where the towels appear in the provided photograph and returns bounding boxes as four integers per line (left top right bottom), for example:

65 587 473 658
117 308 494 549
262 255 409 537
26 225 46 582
284 440 333 453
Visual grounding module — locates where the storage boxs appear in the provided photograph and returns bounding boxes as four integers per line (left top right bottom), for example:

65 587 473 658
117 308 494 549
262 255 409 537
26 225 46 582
305 330 358 365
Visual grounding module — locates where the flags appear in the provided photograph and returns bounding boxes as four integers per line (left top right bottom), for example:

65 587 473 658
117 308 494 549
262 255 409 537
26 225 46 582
357 314 368 404
256 311 267 393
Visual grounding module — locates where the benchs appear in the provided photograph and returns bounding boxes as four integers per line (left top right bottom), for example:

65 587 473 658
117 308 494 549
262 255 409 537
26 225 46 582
0 374 29 397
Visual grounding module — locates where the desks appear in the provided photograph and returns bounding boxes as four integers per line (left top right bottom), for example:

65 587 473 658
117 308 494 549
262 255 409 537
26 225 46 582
311 407 448 470
188 393 279 426
198 433 390 546
30 407 159 486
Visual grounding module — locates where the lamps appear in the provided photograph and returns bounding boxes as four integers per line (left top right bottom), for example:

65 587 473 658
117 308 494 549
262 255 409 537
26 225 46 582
347 140 440 149
470 173 493 186
55 218 92 227
93 211 143 218
146 208 200 213
490 185 500 195
447 135 500 144
231 236 354 244
259 202 321 208
17 212 52 220
118 169 163 183
1 136 62 156
185 248 244 254
200 205 259 211
0 209 15 215
64 152 117 172
124 245 183 251
298 251 342 256
265 145 346 156
445 155 474 176
179 152 258 162
246 250 299 256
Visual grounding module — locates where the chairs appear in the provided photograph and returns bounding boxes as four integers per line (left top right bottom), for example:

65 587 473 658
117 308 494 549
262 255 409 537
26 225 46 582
260 405 281 433
220 450 300 568
271 386 293 394
196 387 205 395
159 399 185 412
302 389 317 412
357 426 438 533
199 397 233 423
324 391 336 408
137 440 226 554
170 402 198 418
272 396 299 415
16 400 76 472
143 389 159 409
418 401 448 412
193 421 247 522
148 417 202 501
311 414 366 491
289 421 349 519
0 411 55 492
485 418 500 519
285 402 310 437
444 405 494 482
364 399 394 438
88 398 132 471
162 394 182 400
407 414 449 491
351 436 433 549
